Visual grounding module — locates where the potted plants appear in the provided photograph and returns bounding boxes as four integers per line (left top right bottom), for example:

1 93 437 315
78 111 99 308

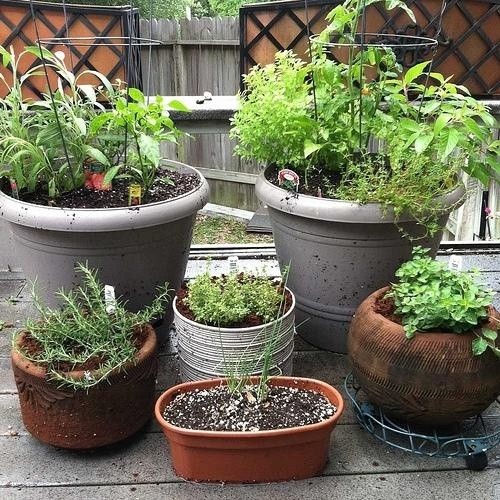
227 0 499 353
348 247 499 430
0 45 210 333
10 258 156 455
153 259 345 485
